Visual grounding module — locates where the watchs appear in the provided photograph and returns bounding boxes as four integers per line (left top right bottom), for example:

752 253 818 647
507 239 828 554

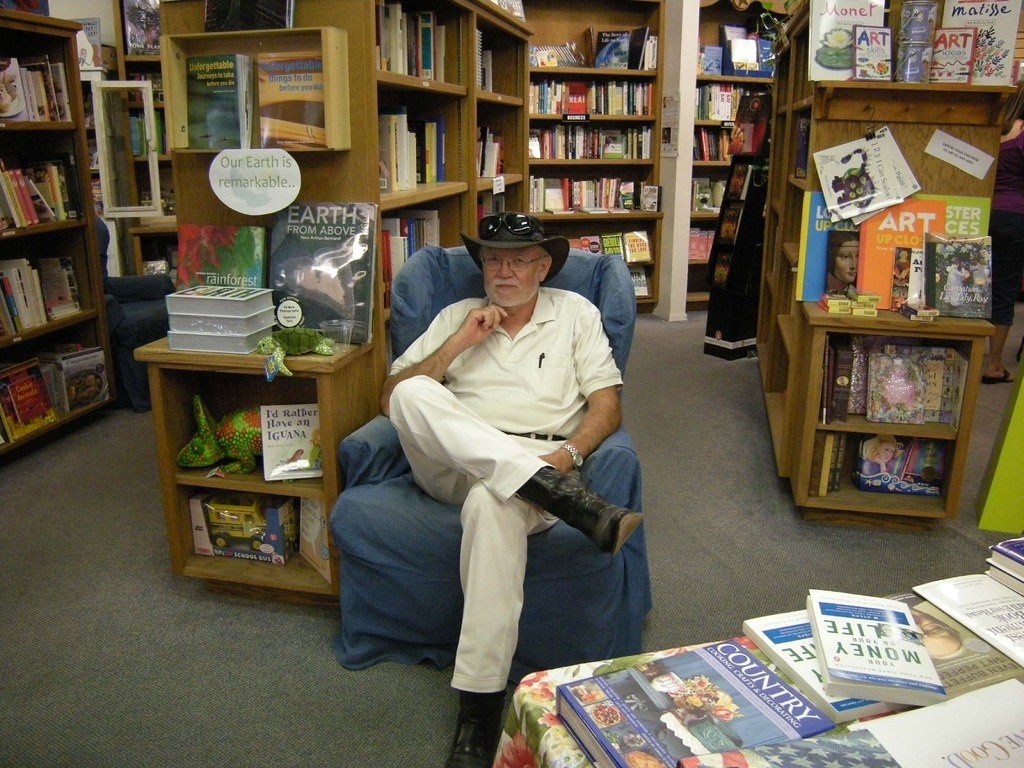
560 445 583 469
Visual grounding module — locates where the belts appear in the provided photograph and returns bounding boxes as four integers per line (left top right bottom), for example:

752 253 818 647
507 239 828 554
503 431 567 441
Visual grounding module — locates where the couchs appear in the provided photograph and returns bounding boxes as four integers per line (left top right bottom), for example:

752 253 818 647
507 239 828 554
94 214 177 414
329 244 655 685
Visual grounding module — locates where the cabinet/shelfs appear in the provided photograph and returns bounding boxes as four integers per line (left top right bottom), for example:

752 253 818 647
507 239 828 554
0 0 1024 610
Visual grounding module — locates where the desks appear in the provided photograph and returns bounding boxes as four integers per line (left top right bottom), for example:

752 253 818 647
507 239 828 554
491 573 1024 768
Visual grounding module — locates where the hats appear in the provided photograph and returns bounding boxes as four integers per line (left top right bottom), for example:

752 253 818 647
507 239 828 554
460 216 570 286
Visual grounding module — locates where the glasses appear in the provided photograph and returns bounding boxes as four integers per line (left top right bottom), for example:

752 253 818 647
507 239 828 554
479 248 546 270
477 213 546 240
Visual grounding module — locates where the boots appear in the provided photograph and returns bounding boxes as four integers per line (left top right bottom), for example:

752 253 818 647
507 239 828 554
516 465 644 555
444 689 506 768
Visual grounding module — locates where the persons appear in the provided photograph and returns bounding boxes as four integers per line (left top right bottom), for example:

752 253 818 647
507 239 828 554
982 80 1024 384
379 214 644 768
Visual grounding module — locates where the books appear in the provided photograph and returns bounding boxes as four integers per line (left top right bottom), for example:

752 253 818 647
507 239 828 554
823 336 969 433
555 535 1024 768
795 114 811 179
0 55 111 445
795 125 992 319
808 0 1022 85
186 51 327 150
689 40 776 258
126 70 179 283
375 0 505 363
491 0 525 21
528 26 661 296
713 253 751 292
808 431 847 497
166 201 378 353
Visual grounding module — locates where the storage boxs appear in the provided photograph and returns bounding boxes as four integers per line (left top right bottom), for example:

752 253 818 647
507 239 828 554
188 488 298 567
300 497 331 585
